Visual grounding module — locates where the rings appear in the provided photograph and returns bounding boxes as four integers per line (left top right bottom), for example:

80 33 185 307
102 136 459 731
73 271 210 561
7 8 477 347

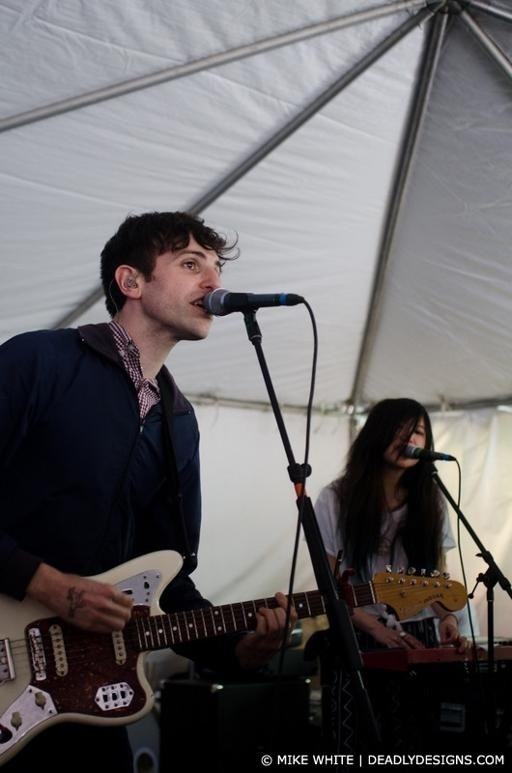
399 631 407 638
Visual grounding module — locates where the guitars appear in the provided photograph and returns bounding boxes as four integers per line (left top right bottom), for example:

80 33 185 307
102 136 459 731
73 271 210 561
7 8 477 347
1 549 468 765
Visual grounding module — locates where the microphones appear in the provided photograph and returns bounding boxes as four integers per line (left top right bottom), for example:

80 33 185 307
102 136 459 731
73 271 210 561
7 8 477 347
405 443 456 464
204 287 303 317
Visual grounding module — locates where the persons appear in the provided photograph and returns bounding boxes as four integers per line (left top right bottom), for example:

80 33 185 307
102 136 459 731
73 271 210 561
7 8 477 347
0 210 304 771
307 395 476 772
